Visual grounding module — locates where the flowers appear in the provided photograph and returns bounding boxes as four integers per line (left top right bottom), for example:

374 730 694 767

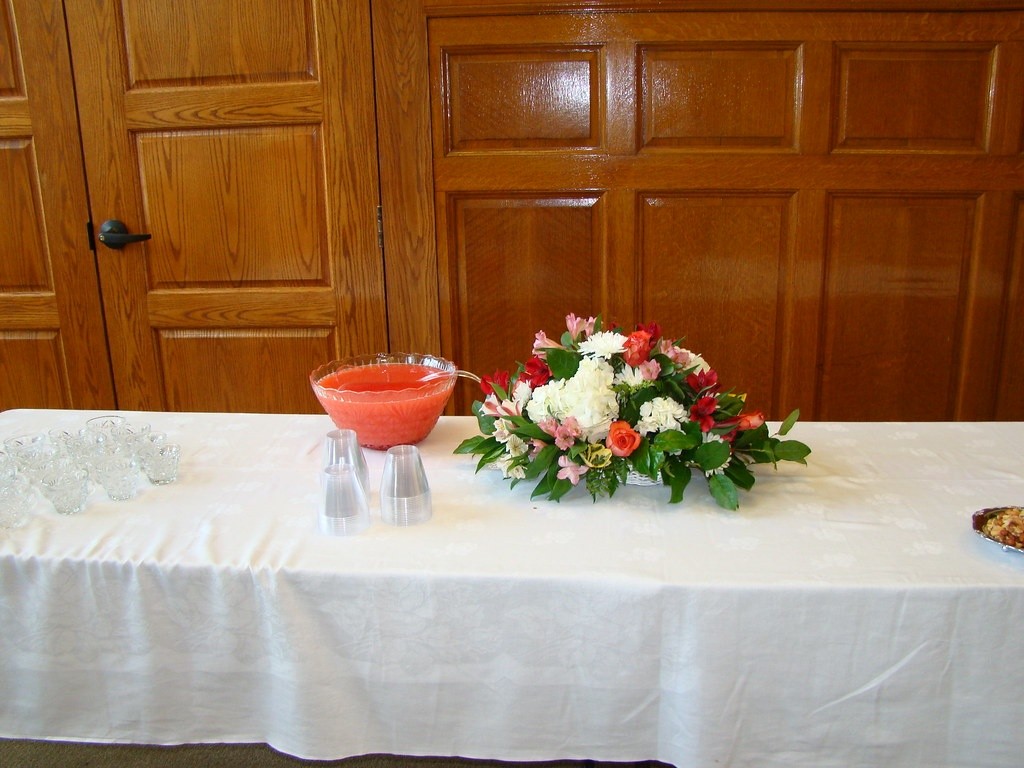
451 306 811 511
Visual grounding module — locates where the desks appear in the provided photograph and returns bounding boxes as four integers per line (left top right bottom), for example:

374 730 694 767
0 409 1024 768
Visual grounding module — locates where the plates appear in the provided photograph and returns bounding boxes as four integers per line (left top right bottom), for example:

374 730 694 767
972 505 1024 554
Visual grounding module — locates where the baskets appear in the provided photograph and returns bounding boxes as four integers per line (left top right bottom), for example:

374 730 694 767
616 464 663 487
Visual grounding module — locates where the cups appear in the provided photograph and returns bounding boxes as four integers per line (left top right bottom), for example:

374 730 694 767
325 430 370 496
317 464 370 539
380 445 432 527
0 416 181 535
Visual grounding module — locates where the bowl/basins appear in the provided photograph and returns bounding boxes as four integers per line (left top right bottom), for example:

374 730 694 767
308 352 458 451
